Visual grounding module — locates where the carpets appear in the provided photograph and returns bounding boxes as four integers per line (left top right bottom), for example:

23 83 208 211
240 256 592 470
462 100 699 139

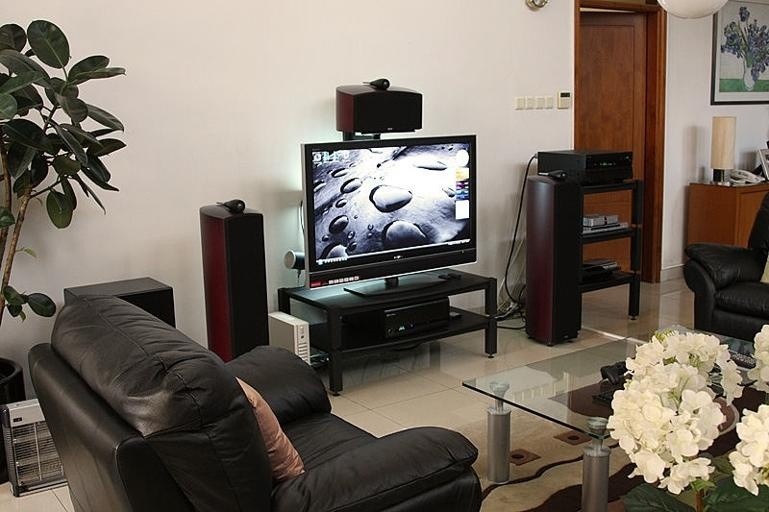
450 383 744 512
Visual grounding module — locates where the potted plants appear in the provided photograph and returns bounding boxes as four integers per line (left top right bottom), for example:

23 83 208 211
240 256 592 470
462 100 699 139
0 18 128 485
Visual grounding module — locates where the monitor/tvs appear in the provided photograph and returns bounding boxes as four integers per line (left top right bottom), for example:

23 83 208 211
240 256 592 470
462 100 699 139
298 135 478 289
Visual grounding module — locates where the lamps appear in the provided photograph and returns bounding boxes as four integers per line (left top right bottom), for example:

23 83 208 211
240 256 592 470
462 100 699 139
710 115 736 186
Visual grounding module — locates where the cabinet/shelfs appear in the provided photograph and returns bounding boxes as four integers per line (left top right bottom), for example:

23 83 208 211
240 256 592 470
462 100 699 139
687 180 768 250
580 177 644 319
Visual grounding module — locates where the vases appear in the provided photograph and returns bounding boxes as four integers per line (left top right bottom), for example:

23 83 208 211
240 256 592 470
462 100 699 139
741 59 756 91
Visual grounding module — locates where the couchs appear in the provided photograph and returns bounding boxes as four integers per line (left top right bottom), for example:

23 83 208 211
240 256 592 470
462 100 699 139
680 191 769 340
28 293 480 512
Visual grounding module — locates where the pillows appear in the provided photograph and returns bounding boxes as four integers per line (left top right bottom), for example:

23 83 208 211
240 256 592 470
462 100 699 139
234 373 306 482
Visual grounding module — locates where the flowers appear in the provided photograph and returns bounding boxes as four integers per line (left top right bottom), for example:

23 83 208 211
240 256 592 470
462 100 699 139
609 324 769 512
719 6 769 81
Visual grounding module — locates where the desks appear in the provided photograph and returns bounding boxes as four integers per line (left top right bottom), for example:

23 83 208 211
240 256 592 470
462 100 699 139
277 268 498 396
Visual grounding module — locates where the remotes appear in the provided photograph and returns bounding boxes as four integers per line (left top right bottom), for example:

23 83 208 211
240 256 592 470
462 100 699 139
728 351 756 369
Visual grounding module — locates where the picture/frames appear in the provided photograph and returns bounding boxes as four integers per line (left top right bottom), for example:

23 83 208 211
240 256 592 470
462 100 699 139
711 2 769 105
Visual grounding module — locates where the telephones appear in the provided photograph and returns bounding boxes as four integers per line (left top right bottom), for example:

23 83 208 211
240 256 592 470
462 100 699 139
729 169 765 184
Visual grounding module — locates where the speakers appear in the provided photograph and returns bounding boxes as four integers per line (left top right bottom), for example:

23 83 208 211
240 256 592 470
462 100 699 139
327 78 423 140
199 200 272 365
528 173 582 346
